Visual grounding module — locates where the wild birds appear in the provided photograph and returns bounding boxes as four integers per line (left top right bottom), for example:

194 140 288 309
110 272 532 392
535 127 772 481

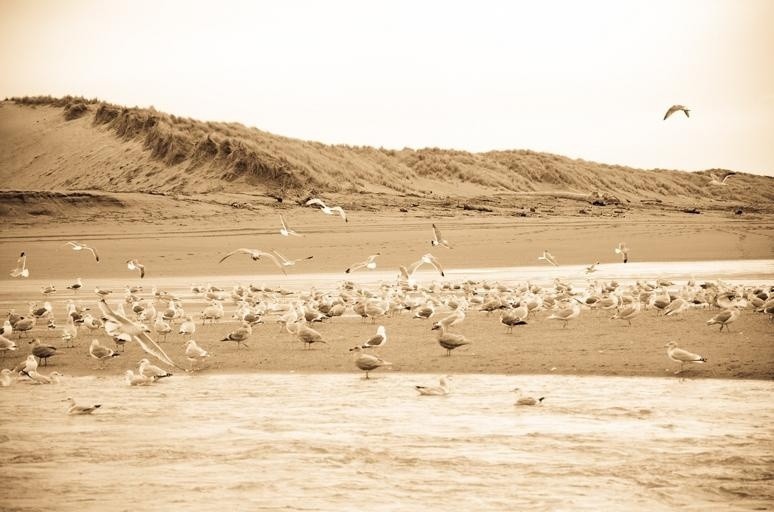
0 242 774 397
430 222 456 250
60 397 100 415
509 387 546 406
664 105 690 120
705 173 736 187
305 198 348 223
279 213 306 238
